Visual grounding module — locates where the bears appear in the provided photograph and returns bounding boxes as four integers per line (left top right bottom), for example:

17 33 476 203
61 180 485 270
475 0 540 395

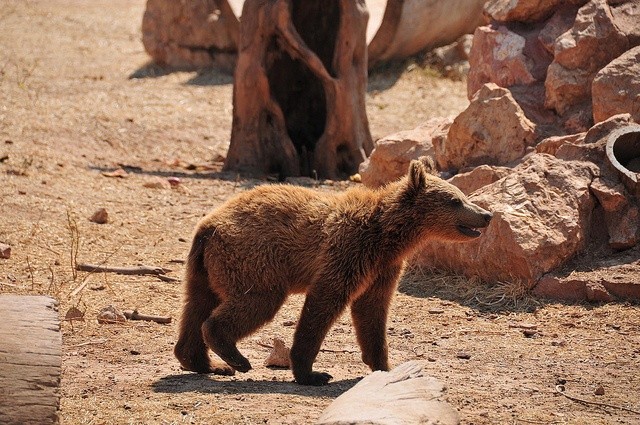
174 154 493 386
